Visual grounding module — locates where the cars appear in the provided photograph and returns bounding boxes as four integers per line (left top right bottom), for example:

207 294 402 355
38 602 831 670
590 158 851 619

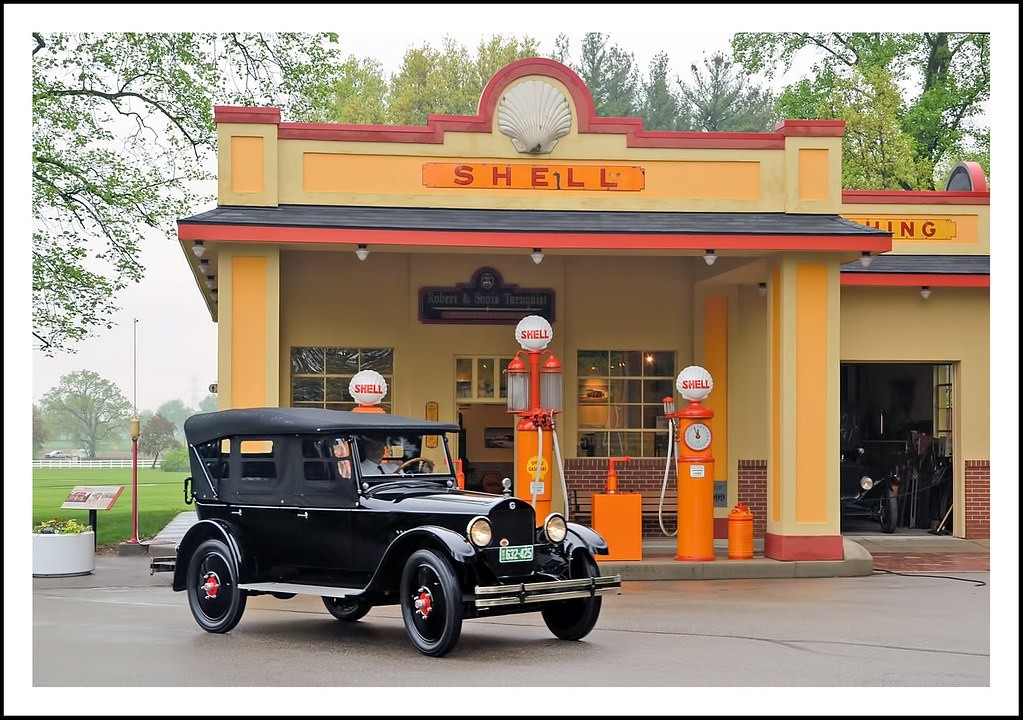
149 407 622 657
840 441 899 533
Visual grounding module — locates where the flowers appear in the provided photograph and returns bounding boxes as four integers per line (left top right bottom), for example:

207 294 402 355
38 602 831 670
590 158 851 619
33 516 93 534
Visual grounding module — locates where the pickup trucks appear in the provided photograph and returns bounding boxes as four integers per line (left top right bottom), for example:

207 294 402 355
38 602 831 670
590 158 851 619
45 450 71 457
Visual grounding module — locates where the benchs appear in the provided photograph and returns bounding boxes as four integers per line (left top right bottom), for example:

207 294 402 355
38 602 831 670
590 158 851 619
570 487 677 524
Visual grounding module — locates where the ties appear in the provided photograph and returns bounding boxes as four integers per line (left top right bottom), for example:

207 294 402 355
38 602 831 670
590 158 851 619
377 466 385 474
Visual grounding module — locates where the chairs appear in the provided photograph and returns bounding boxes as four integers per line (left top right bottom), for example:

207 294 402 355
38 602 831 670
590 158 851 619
265 441 329 480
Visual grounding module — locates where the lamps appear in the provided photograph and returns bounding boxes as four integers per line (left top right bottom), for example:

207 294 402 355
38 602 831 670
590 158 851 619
859 251 872 268
508 351 528 414
204 276 216 289
920 286 931 299
209 290 218 302
540 351 562 413
704 250 717 265
198 259 209 273
530 249 544 264
191 240 205 258
355 244 369 260
758 283 766 295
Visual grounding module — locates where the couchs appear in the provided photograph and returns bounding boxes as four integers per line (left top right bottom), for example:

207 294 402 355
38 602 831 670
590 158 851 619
482 473 505 494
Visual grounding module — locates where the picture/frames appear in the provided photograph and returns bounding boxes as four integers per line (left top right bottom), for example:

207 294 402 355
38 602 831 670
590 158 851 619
484 427 514 449
457 380 472 409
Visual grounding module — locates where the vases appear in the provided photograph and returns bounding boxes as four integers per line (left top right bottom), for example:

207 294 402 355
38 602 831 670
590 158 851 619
32 531 95 578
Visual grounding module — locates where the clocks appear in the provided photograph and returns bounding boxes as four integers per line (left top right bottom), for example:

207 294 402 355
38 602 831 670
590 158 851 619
684 422 712 451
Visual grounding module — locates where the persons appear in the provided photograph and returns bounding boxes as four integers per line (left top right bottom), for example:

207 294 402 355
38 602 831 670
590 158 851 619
360 438 435 476
